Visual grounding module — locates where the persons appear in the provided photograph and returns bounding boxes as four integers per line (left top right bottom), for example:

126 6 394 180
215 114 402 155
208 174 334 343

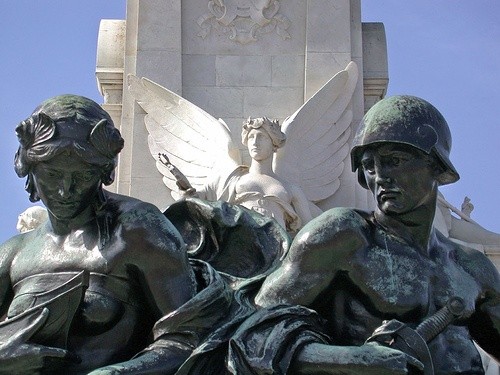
0 95 500 375
